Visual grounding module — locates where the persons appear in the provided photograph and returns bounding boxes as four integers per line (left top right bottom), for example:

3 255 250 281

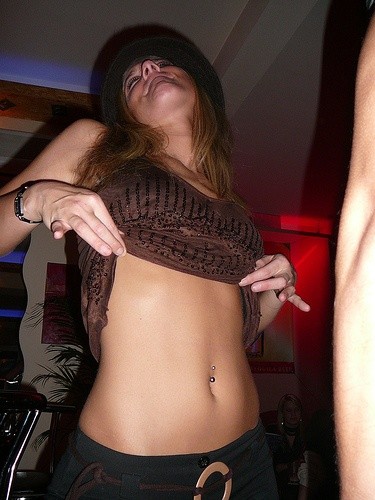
0 14 375 500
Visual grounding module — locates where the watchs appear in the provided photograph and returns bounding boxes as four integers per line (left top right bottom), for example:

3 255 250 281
14 181 43 224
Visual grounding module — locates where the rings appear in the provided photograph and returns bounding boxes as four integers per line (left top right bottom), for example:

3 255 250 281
277 272 291 289
